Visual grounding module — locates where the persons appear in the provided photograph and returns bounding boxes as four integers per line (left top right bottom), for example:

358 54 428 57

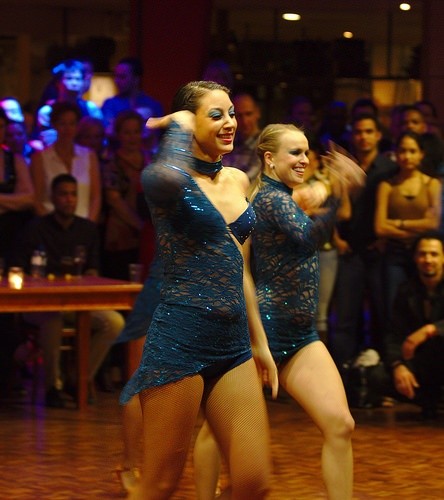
31 103 101 221
77 118 110 222
0 99 42 397
373 234 444 416
374 129 444 315
35 174 125 410
193 123 355 500
119 80 279 500
292 88 444 346
102 58 163 156
102 108 154 281
37 61 105 146
218 91 270 178
332 114 402 413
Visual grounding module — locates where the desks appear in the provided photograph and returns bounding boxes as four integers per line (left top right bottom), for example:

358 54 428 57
0 274 145 413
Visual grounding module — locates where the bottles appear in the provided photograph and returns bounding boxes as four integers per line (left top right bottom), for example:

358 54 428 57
31 249 48 281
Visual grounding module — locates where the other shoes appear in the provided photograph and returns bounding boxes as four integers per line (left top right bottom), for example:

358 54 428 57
98 371 115 392
422 402 436 418
45 389 64 408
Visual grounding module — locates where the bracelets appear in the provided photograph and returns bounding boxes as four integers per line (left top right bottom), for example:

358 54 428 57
392 362 401 368
